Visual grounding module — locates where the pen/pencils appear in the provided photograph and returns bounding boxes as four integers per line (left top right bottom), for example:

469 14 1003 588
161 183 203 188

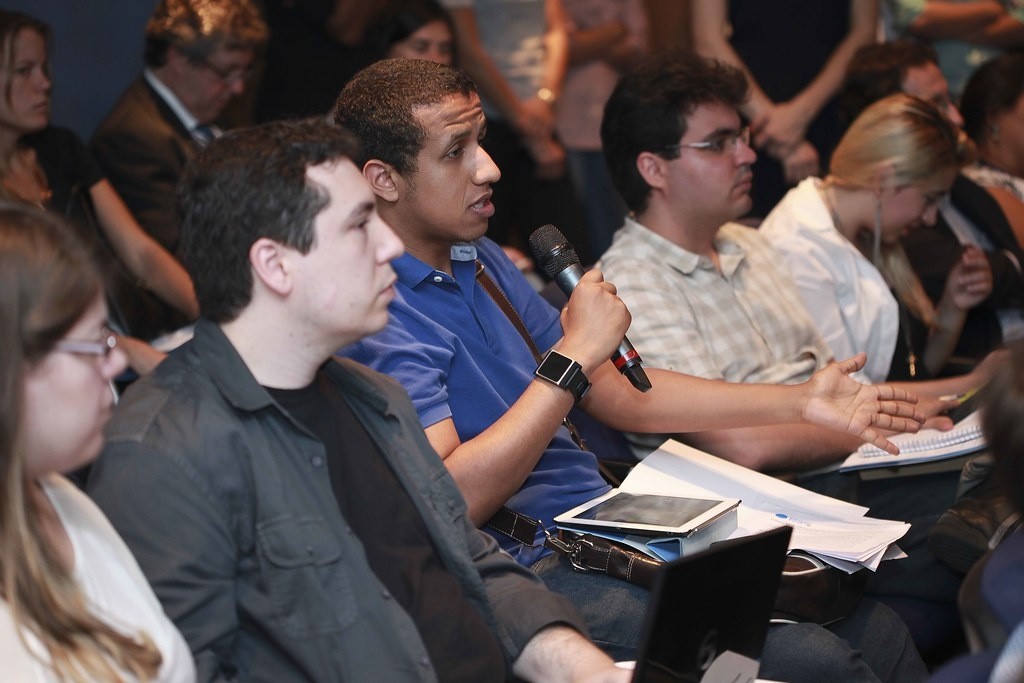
939 393 964 400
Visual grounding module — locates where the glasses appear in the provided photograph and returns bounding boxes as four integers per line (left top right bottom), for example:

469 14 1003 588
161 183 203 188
653 123 752 156
46 320 119 366
190 48 252 85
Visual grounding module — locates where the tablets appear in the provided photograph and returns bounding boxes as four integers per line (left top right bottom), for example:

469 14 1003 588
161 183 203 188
553 488 742 539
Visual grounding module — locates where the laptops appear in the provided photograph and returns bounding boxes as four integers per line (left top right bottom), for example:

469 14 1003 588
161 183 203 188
628 525 794 683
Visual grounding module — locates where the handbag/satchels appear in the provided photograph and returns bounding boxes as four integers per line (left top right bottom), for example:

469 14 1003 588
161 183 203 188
553 525 875 625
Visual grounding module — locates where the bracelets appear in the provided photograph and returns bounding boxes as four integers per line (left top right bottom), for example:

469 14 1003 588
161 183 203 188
538 88 558 108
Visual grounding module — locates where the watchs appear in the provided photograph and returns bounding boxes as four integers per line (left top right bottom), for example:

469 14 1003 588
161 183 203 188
533 349 592 402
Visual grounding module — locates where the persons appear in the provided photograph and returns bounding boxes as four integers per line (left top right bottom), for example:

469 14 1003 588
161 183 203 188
0 200 194 683
597 51 1024 683
329 0 1024 256
332 58 926 663
0 11 201 324
86 0 269 254
84 118 634 683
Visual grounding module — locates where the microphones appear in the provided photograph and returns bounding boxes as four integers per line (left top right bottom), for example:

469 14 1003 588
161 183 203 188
527 223 653 393
